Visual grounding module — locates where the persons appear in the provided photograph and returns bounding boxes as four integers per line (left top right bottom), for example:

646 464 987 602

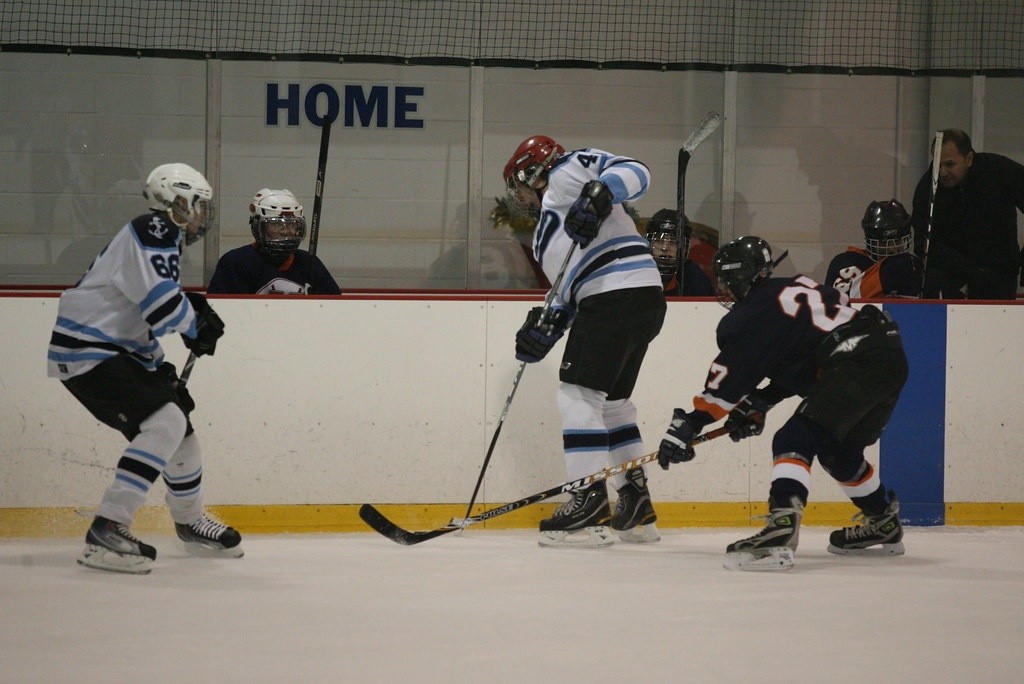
823 198 965 299
658 235 909 552
206 188 341 294
503 135 667 531
911 128 1024 299
642 208 715 296
47 162 245 562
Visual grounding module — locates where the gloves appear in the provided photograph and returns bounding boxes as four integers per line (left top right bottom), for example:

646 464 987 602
157 361 194 415
564 181 615 249
725 390 776 442
515 306 569 362
938 272 976 300
879 253 924 295
659 406 696 469
182 290 224 357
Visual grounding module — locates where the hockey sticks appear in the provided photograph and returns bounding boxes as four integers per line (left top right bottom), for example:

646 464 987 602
675 111 724 296
918 130 944 296
72 351 198 517
359 412 761 545
304 115 332 296
413 182 603 538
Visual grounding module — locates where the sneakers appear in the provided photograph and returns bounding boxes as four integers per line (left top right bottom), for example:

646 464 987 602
538 469 614 549
725 494 804 571
175 509 244 558
610 467 661 544
77 515 156 574
826 490 905 557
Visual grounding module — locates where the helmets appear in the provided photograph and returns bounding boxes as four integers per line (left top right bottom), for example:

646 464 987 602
862 199 912 262
643 209 692 279
143 162 213 227
503 135 565 191
711 236 772 310
249 188 305 260
